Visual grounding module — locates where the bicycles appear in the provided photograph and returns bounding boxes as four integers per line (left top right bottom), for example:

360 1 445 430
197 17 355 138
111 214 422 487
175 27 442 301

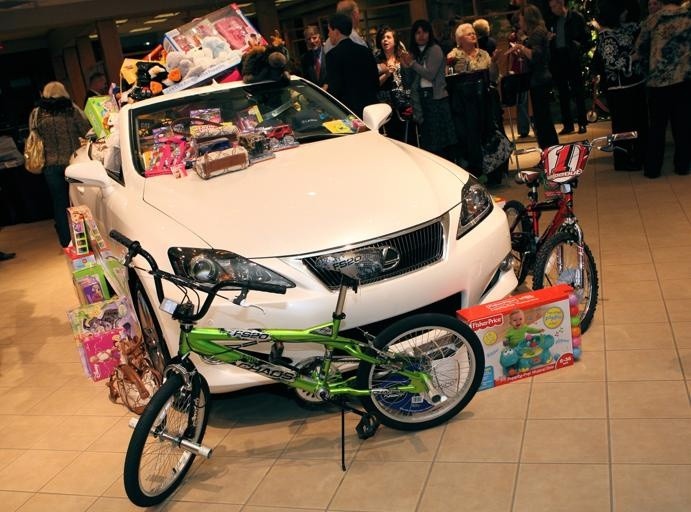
105 224 490 507
503 131 640 338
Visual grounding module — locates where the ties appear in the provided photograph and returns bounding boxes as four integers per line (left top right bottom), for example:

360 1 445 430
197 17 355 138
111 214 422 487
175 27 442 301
313 53 321 82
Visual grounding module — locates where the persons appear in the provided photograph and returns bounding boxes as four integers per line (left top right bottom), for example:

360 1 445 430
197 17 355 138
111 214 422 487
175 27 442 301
84 72 106 109
503 309 545 348
298 1 529 168
29 81 90 249
585 1 691 178
514 5 559 168
549 0 592 135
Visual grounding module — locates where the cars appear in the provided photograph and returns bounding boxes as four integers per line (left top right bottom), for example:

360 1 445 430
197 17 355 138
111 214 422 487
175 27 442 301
62 72 521 415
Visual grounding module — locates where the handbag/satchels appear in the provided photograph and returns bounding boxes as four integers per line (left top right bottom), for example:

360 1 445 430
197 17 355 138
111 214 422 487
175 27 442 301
106 364 163 415
479 129 514 175
24 107 46 175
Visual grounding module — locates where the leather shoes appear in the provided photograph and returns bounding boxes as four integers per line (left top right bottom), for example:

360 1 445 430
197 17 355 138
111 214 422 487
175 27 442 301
0 252 16 261
579 124 586 134
559 127 574 135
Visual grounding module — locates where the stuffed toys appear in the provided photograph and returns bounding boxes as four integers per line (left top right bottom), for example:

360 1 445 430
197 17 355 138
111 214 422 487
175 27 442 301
108 1 290 103
